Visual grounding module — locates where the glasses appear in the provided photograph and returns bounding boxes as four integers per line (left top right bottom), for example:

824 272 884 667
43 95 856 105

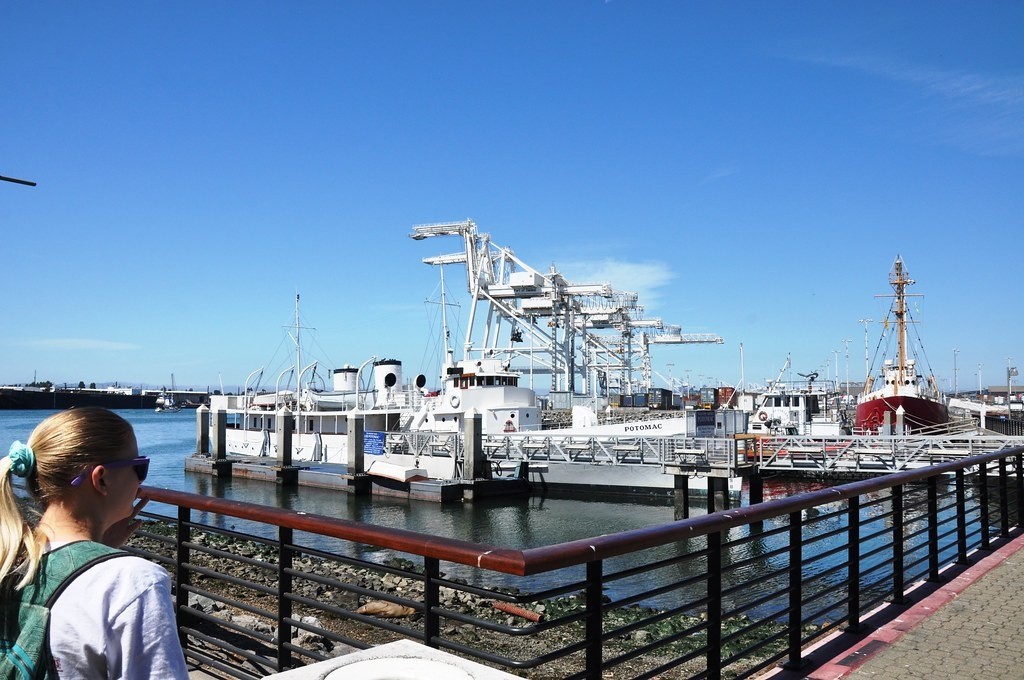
70 455 150 486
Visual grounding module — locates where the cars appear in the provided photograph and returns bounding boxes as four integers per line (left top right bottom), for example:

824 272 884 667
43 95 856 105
1010 393 1024 402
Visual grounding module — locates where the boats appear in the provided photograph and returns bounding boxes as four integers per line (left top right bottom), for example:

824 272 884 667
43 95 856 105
206 284 541 474
855 256 950 440
179 399 211 408
749 352 855 438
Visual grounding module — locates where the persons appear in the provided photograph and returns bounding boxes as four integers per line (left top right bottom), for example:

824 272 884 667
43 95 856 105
0 408 190 680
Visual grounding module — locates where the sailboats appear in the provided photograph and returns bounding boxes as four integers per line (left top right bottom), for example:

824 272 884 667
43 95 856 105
155 372 181 413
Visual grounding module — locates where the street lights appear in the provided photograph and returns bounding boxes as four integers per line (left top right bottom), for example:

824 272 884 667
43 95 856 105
1004 356 1014 402
666 363 674 389
698 374 703 386
686 369 693 395
952 348 960 397
978 363 985 396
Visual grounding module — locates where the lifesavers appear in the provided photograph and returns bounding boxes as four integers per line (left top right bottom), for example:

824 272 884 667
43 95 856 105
758 411 767 421
450 395 460 409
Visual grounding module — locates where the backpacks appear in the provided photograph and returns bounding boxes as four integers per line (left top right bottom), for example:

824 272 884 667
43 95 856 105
0 540 130 680
